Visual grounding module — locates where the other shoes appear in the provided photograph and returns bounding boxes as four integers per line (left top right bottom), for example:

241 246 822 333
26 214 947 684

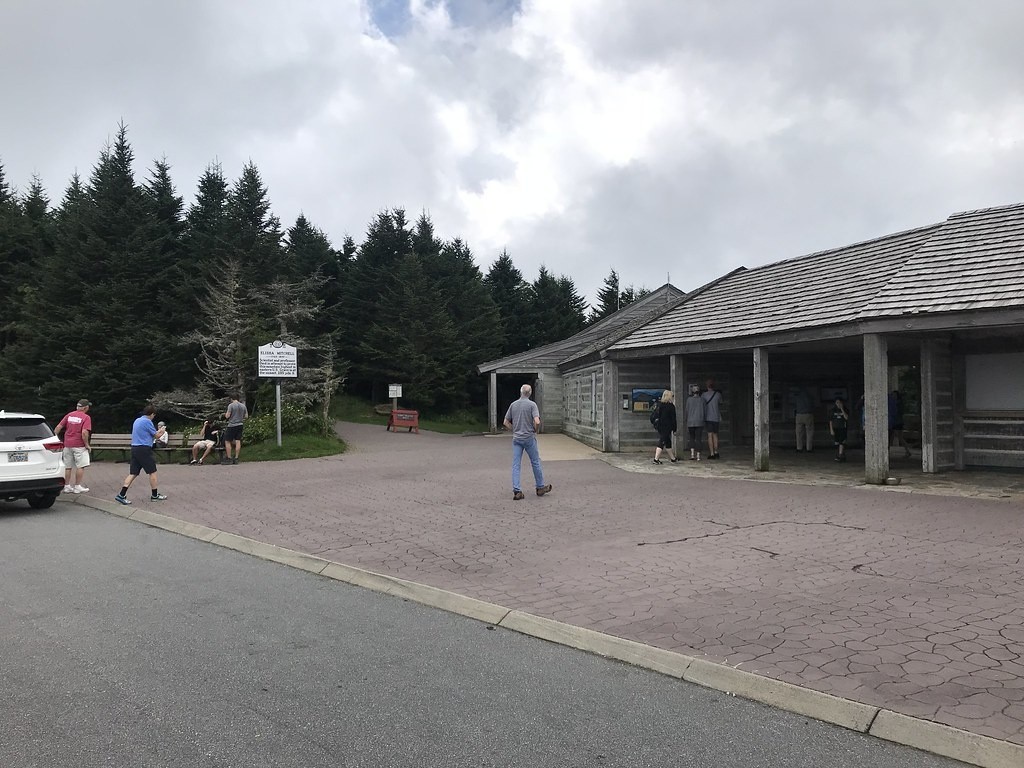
834 456 841 461
233 458 239 464
198 459 203 466
707 455 716 459
795 450 803 454
807 450 812 454
905 452 911 457
714 453 719 459
191 460 197 465
653 459 662 464
671 458 680 463
689 456 694 459
221 458 232 464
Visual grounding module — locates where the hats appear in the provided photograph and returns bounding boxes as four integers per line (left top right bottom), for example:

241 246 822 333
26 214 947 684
77 399 93 407
691 386 699 392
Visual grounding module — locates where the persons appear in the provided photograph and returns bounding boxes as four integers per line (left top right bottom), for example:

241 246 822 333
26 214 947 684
829 397 849 462
795 387 815 452
887 390 911 459
54 399 91 494
701 379 723 460
115 406 167 505
153 422 169 448
504 384 552 500
686 386 707 461
190 418 220 466
653 390 681 465
221 393 247 465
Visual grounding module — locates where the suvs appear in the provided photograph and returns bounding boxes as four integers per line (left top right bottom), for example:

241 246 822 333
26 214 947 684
0 410 66 508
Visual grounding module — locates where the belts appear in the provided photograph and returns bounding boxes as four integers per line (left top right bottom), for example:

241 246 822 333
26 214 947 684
796 411 812 414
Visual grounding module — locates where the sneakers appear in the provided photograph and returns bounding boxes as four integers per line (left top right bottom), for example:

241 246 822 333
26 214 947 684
537 485 552 495
151 493 167 502
115 494 132 504
63 487 74 493
513 491 524 499
73 485 89 493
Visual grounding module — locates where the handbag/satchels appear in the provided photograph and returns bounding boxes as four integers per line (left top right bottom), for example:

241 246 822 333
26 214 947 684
650 406 659 429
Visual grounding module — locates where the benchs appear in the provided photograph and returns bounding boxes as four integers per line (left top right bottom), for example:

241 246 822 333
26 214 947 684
91 432 227 461
